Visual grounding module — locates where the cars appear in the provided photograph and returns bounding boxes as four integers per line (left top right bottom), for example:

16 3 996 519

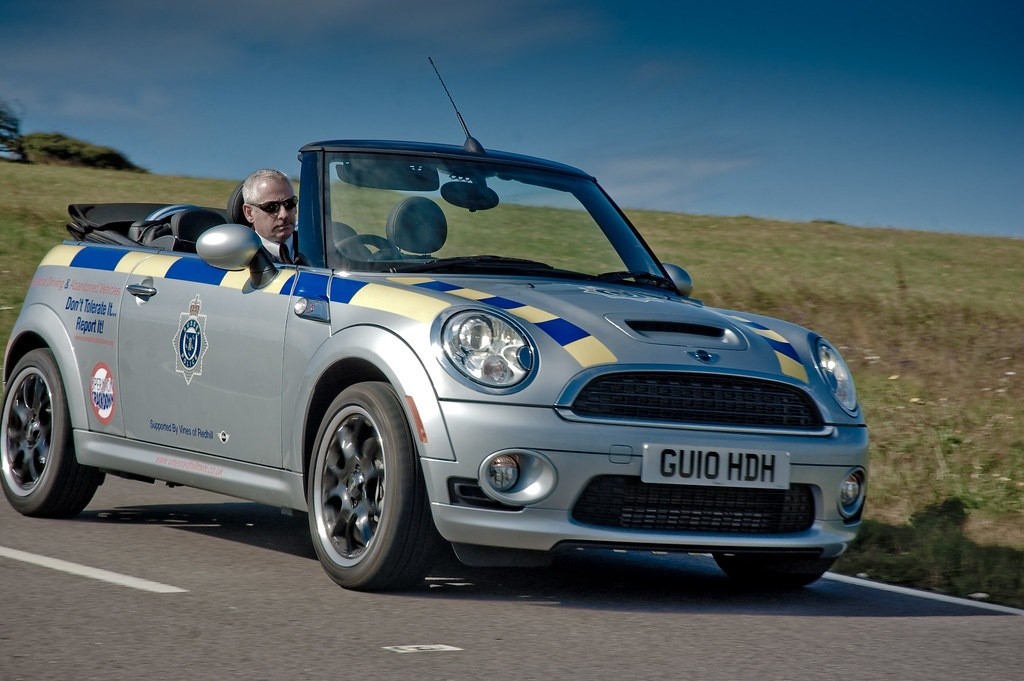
0 139 870 589
0 148 22 159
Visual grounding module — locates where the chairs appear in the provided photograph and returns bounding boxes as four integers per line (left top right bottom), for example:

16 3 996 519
171 208 229 243
227 180 253 228
386 197 447 253
331 221 372 255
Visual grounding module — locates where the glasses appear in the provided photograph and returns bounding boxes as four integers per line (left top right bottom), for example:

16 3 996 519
250 195 298 213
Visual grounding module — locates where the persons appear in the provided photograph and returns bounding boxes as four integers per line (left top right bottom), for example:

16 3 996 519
242 169 306 266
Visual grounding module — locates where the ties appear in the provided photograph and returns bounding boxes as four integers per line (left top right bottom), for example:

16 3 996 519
279 242 293 265
